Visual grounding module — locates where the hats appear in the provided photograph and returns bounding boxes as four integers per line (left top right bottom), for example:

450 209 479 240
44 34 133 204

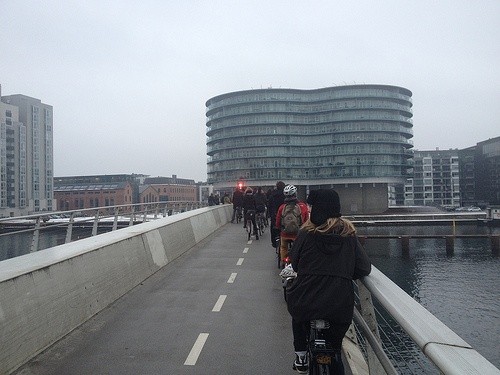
306 189 339 225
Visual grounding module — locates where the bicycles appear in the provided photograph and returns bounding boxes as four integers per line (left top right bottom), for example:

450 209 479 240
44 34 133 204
233 205 294 301
282 274 340 375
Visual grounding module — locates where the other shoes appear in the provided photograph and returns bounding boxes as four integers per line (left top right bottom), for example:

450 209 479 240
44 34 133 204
294 354 309 373
244 225 246 228
256 236 259 240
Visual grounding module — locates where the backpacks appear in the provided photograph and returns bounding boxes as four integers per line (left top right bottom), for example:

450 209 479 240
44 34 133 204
280 202 302 234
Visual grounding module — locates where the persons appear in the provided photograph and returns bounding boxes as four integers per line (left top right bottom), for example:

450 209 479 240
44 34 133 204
268 181 285 248
231 186 274 240
286 188 371 372
275 184 309 270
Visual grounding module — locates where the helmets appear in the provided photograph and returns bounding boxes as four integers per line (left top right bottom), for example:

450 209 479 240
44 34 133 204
283 184 297 196
